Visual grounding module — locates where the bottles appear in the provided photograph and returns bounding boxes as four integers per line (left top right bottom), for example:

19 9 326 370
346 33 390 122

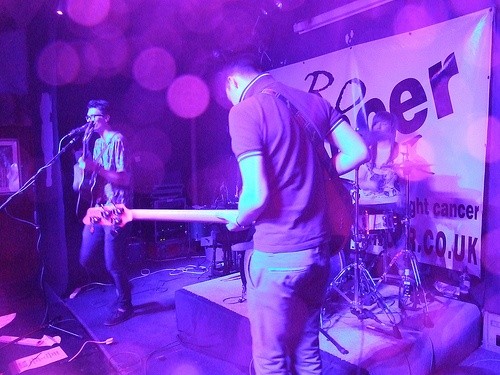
460 266 471 303
398 269 417 311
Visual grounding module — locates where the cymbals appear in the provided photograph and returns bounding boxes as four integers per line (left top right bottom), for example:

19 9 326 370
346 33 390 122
357 129 392 145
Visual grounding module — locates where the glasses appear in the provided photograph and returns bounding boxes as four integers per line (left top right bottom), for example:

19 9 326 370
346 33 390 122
85 115 104 123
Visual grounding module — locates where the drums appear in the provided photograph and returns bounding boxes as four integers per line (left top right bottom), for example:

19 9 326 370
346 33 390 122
353 200 400 230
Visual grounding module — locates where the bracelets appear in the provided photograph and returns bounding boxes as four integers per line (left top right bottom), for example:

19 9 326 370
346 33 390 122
235 216 252 230
95 165 102 173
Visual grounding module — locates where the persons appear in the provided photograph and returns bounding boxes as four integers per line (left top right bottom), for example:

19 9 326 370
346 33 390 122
220 55 371 375
78 99 135 326
353 111 408 278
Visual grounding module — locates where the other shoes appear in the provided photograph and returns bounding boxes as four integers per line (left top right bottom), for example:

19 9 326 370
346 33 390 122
104 305 135 326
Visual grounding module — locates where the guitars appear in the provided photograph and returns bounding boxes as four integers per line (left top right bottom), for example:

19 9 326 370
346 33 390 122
83 178 352 257
72 120 102 221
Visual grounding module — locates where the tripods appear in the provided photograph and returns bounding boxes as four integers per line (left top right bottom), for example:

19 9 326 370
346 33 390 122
322 165 435 341
0 135 84 353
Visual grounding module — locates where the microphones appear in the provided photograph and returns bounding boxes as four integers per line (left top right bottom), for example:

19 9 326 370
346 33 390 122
67 123 93 137
